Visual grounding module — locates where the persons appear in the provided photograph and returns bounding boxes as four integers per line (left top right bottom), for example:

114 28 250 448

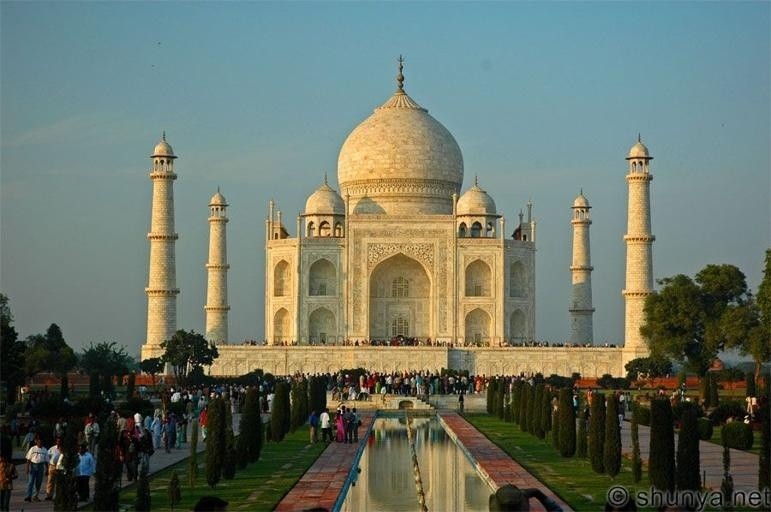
501 370 691 429
746 392 760 420
194 495 229 511
214 333 624 348
0 445 154 512
0 368 501 445
657 494 699 511
606 495 638 512
